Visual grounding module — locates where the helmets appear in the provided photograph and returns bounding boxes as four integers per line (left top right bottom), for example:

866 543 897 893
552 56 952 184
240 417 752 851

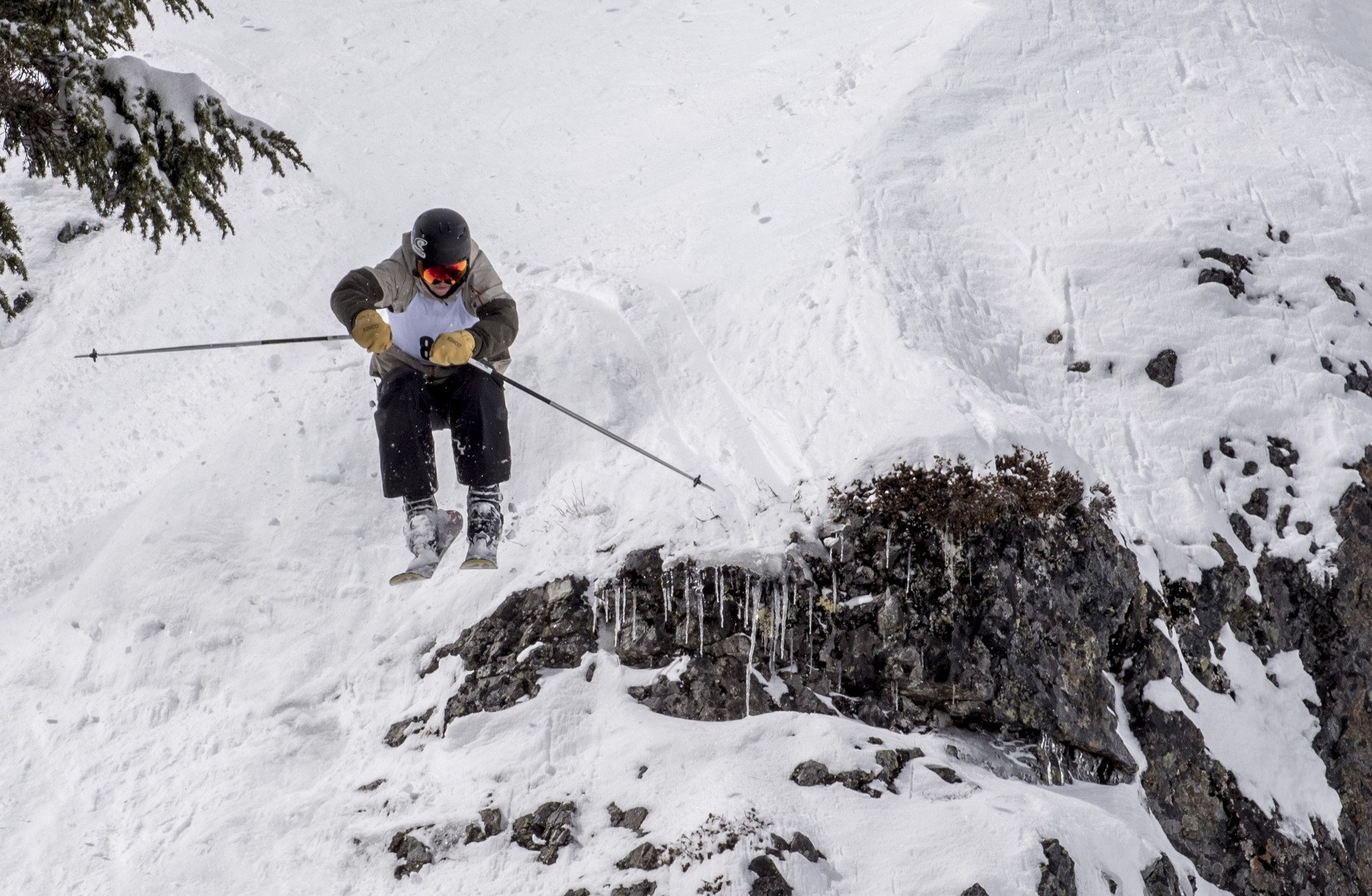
411 208 472 282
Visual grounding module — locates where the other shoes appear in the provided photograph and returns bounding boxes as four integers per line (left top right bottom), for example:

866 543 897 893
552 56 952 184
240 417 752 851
466 483 504 545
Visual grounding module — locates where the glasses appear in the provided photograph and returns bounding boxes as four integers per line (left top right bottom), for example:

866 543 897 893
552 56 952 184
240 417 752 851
422 257 468 286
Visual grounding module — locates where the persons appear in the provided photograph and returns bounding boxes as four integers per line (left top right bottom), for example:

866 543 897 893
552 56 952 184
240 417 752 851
331 207 518 557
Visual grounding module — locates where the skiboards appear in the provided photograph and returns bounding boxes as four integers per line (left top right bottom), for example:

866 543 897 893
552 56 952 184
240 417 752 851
387 510 504 587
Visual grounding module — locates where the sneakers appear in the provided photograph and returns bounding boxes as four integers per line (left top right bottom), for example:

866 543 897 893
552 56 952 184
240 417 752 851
401 495 438 552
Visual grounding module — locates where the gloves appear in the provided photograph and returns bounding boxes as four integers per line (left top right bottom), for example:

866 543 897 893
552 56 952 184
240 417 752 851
428 329 477 366
349 310 393 355
